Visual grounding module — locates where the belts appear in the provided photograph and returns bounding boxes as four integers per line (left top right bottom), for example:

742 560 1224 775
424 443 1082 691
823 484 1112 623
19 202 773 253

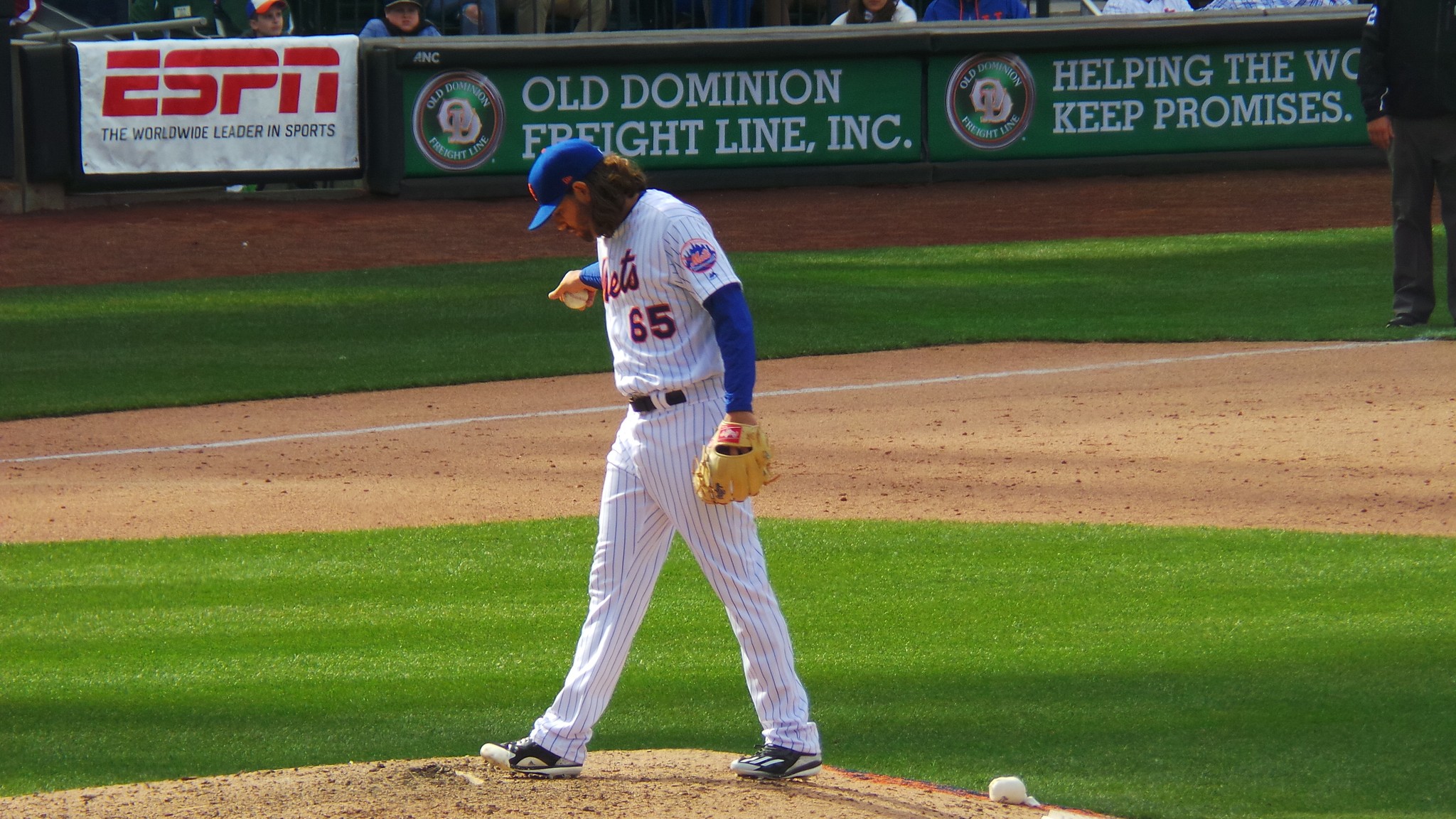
629 389 687 412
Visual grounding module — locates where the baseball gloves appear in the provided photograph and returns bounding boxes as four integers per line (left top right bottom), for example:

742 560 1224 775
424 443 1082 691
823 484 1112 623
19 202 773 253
692 421 776 505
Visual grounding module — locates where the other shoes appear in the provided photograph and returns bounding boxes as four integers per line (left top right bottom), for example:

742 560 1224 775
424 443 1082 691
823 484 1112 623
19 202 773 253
1386 314 1425 328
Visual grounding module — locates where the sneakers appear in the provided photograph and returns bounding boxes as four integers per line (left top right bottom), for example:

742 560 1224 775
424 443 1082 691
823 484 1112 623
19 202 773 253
730 740 822 780
481 736 582 779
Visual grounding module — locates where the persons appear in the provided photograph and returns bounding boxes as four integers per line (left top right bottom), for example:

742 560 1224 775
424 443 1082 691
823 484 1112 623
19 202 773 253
128 0 294 41
1358 0 1455 328
703 0 750 28
480 139 825 781
831 0 918 25
359 0 613 40
923 0 1031 23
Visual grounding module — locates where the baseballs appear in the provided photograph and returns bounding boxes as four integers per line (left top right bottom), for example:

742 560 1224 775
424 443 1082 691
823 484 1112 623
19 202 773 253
562 287 588 310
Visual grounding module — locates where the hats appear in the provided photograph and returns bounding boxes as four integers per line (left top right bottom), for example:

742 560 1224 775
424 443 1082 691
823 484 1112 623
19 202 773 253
527 140 603 231
385 0 423 8
247 0 287 19
